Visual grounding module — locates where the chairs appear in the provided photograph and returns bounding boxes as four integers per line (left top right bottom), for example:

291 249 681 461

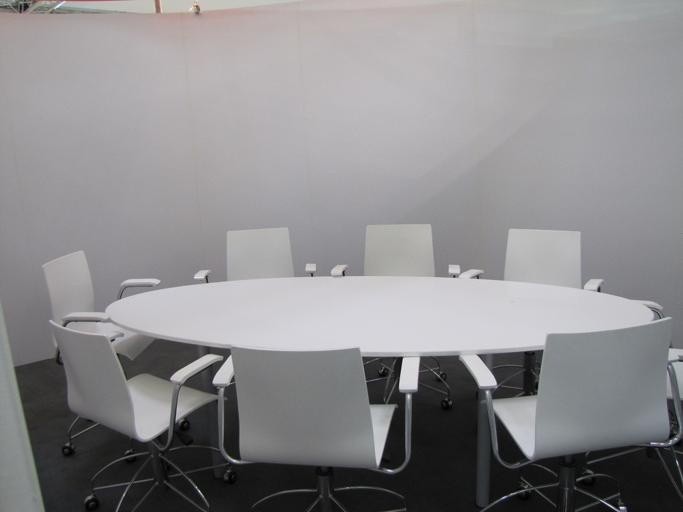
210 346 421 512
193 226 317 283
39 249 161 454
459 315 671 512
48 318 228 511
459 227 604 396
641 297 683 403
329 223 460 412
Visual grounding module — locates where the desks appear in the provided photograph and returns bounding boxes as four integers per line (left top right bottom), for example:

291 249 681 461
104 274 655 510
